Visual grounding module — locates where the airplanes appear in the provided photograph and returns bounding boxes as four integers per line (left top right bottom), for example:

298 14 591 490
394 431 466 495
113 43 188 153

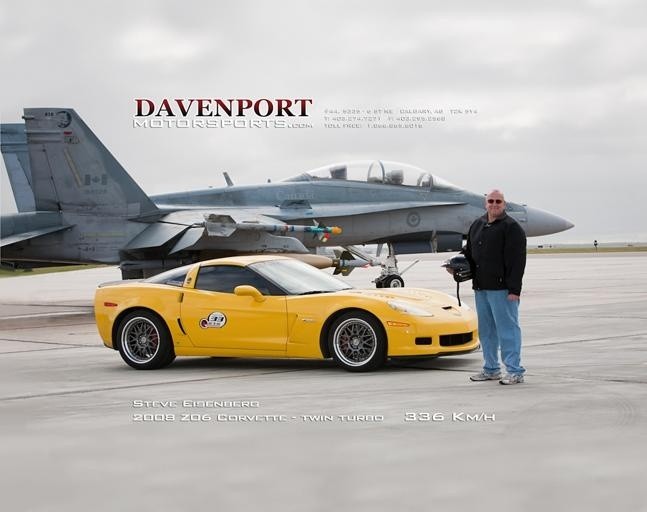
0 106 581 290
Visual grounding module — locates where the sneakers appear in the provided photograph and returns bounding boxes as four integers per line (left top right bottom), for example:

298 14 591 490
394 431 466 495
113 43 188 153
499 375 524 385
470 372 503 381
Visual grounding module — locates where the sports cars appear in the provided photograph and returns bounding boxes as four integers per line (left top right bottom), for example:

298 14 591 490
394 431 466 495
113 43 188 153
89 253 482 373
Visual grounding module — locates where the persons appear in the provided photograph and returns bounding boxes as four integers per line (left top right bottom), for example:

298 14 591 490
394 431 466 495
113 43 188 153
444 189 527 385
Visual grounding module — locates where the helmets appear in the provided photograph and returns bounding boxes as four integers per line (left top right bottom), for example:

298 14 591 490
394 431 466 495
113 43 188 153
441 255 472 282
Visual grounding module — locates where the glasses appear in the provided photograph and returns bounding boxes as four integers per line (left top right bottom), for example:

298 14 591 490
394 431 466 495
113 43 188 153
488 199 502 204
